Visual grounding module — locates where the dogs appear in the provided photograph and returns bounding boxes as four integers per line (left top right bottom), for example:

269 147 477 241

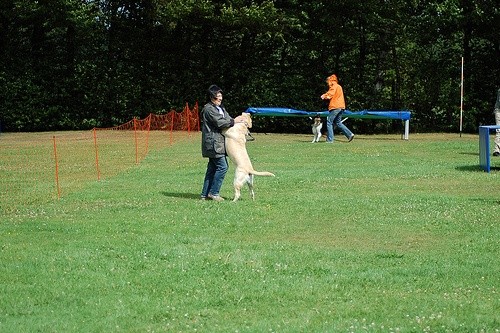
221 112 276 203
310 117 326 144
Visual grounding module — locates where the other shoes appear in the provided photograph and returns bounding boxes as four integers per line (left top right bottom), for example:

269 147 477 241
325 140 336 144
201 193 225 202
347 133 355 143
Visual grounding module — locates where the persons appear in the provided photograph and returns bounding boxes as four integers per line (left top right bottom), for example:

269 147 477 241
320 74 355 145
198 84 244 201
